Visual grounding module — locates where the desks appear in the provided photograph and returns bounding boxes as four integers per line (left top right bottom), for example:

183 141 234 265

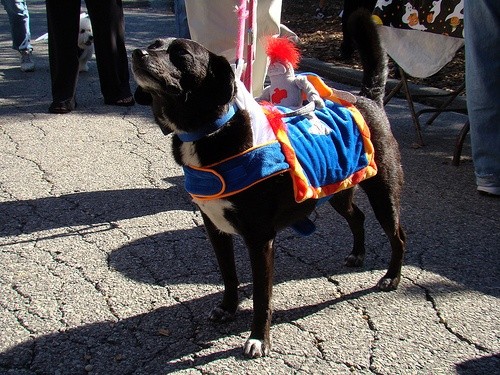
339 0 470 166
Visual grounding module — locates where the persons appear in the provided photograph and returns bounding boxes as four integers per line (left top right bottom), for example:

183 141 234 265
1 0 192 113
463 0 500 197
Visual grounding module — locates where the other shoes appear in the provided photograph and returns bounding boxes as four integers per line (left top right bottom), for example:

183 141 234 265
113 96 135 107
20 49 35 71
52 98 77 114
477 185 500 195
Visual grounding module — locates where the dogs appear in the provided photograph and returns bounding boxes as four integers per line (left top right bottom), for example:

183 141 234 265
78 18 93 72
132 38 406 358
